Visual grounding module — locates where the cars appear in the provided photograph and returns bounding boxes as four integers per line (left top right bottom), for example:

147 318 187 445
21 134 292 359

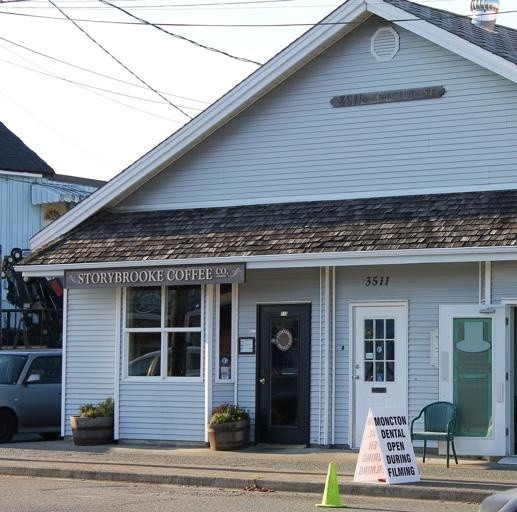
129 346 200 376
0 348 62 443
271 334 298 420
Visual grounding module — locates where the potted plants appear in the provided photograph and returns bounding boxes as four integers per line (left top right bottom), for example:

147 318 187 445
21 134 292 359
208 402 250 451
69 397 114 446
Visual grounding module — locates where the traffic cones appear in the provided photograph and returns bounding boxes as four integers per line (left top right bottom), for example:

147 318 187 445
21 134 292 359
315 460 346 508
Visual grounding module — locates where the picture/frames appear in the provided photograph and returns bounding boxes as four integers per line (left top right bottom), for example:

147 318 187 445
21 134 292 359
238 337 255 354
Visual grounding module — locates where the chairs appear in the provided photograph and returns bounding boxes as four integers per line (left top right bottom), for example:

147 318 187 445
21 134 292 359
410 401 458 468
